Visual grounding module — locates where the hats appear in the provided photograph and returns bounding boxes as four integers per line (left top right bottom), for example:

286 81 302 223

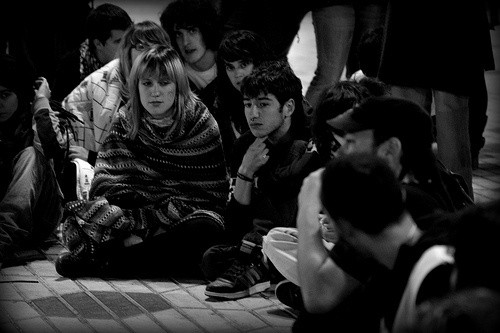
325 96 434 146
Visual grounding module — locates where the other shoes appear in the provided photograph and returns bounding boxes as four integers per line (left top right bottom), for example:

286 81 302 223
54 251 108 279
275 280 303 310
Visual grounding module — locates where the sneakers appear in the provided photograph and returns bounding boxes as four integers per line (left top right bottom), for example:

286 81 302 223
205 261 271 299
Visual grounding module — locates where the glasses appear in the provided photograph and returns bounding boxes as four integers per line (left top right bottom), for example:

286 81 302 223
131 43 146 51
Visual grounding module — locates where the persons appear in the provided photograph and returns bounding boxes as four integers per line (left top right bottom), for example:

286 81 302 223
56 45 233 279
159 0 228 106
65 3 138 88
61 20 176 203
0 54 66 265
208 0 500 333
